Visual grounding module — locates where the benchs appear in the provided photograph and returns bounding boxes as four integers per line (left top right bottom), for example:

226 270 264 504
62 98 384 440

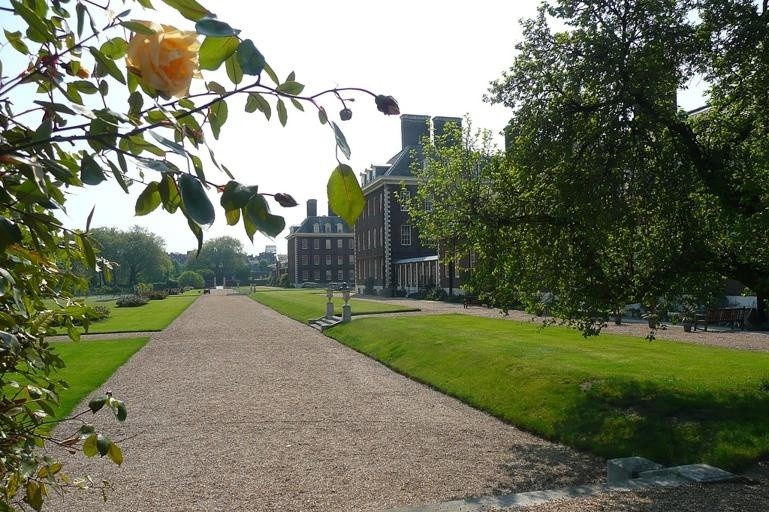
694 306 745 331
463 293 490 309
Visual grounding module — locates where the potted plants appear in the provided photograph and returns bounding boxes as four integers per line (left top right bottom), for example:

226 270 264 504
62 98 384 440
681 305 695 331
612 302 624 324
640 306 659 328
